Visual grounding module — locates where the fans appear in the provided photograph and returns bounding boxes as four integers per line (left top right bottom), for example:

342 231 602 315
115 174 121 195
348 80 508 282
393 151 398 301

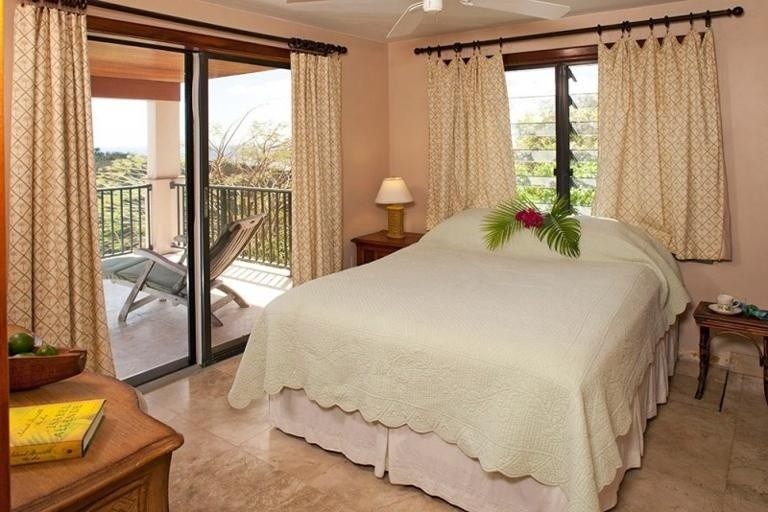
384 0 571 41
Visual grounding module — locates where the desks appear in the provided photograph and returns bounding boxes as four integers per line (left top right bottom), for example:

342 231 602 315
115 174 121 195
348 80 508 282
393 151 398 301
692 301 768 405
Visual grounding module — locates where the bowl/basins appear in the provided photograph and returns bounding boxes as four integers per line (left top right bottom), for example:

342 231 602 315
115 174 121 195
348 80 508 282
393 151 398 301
9 347 87 392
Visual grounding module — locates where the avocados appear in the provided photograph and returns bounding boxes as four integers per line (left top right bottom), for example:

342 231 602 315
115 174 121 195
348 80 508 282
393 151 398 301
9 334 35 354
12 352 37 357
36 345 58 356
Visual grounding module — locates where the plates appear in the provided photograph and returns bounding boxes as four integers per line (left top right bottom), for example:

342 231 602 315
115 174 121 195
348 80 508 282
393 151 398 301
709 303 742 315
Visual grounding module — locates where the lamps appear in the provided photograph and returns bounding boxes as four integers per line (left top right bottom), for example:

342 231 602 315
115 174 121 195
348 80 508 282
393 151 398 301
374 176 415 240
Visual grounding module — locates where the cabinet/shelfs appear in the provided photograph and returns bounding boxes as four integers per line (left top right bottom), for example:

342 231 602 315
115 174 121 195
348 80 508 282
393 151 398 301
5 367 186 512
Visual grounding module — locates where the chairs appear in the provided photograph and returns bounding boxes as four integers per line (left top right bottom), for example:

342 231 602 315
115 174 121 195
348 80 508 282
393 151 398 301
101 212 269 327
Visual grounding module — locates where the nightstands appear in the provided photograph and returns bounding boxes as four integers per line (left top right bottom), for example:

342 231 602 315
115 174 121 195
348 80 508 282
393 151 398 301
350 229 425 267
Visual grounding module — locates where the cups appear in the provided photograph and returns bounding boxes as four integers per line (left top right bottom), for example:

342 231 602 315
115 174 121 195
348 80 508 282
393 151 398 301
717 294 740 313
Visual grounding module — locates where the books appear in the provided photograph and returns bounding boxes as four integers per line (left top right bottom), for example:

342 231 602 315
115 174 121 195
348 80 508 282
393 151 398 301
8 399 107 466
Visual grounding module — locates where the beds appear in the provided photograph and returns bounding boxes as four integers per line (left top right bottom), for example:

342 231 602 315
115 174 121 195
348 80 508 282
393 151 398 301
231 206 694 511
